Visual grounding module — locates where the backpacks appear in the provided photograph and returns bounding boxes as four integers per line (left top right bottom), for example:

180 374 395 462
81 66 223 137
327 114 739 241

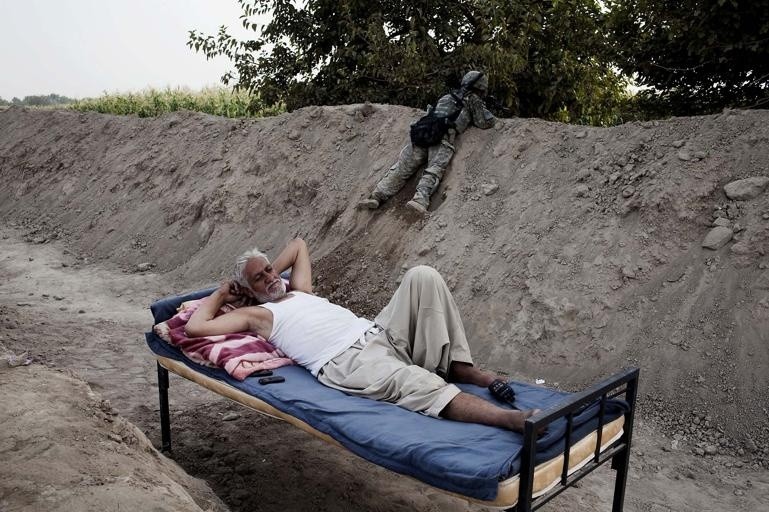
409 115 448 149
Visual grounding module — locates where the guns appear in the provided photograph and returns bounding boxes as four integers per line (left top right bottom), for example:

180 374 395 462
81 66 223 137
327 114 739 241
443 71 513 118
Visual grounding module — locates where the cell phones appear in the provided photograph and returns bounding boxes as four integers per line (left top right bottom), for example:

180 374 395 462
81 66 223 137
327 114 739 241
259 376 285 385
248 370 273 377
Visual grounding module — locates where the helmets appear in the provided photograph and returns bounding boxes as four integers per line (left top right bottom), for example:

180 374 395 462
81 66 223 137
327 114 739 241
461 71 488 91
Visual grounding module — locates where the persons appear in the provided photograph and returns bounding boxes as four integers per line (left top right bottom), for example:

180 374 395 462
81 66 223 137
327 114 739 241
358 71 498 218
184 238 547 438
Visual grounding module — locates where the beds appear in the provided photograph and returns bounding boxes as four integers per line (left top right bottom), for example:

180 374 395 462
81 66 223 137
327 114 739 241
143 271 640 512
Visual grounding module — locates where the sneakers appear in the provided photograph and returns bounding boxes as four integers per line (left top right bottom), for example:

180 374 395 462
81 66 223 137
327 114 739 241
407 201 426 213
358 198 379 209
